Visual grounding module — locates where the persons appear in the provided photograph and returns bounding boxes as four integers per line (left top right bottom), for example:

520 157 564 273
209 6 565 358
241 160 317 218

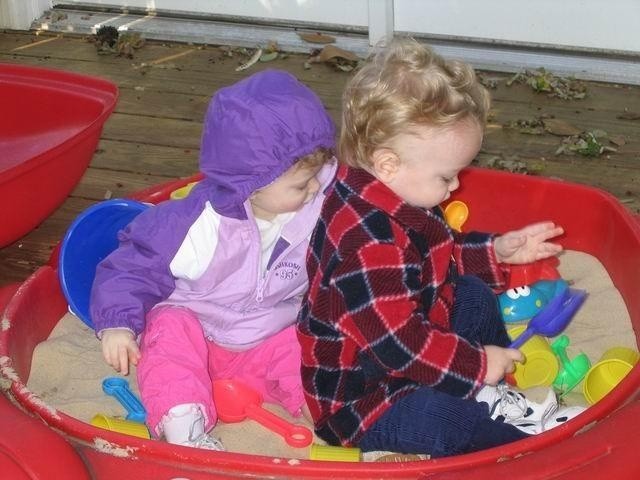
89 68 337 453
295 32 597 457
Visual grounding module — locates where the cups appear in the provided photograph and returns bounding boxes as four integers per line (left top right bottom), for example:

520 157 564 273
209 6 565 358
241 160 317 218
582 346 640 410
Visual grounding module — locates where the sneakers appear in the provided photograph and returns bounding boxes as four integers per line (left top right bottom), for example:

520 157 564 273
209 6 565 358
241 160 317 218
474 383 584 437
161 410 224 453
